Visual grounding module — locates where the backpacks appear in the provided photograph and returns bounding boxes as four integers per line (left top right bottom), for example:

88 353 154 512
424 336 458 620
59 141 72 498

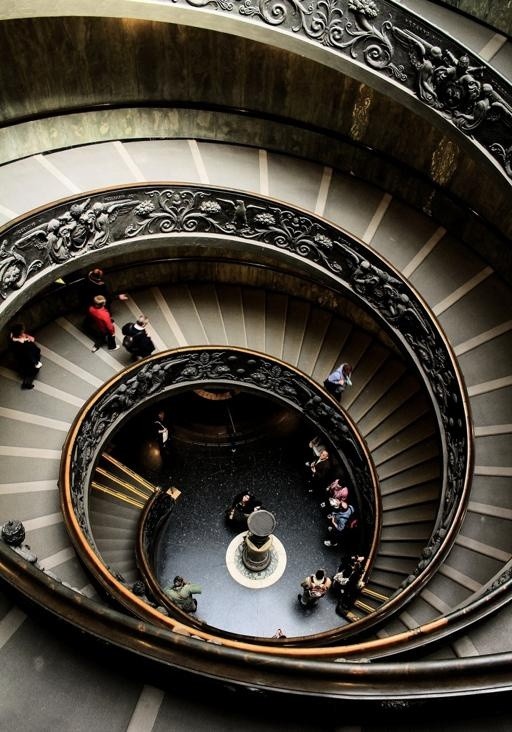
122 324 143 351
309 577 327 600
340 506 359 528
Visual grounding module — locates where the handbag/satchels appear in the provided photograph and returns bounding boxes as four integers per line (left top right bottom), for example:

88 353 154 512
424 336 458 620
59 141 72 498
183 598 196 612
162 430 168 443
324 378 340 394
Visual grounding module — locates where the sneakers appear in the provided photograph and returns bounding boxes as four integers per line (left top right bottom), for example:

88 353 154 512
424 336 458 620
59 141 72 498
298 594 318 608
22 383 34 389
92 344 120 352
324 526 339 547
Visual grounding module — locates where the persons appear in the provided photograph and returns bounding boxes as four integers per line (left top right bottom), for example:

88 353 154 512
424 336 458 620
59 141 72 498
87 294 121 353
323 362 353 403
225 491 266 522
297 430 365 611
151 411 170 449
163 575 202 614
8 320 43 391
121 314 155 365
85 267 128 323
